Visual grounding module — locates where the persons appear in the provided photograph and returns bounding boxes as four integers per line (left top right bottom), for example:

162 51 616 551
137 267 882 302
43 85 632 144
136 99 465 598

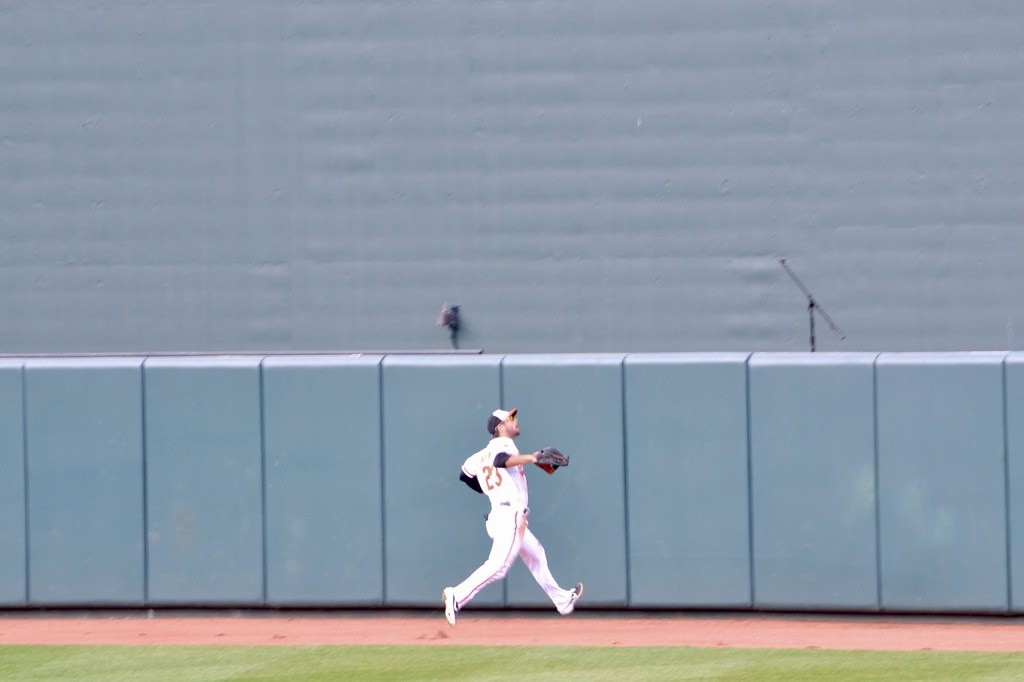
447 304 460 349
442 408 584 626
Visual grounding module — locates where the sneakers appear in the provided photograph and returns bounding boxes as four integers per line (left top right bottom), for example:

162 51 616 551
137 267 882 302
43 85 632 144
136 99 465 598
559 583 583 615
444 587 459 625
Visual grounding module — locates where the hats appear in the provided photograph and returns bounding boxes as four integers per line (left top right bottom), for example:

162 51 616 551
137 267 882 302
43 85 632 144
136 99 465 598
488 409 517 433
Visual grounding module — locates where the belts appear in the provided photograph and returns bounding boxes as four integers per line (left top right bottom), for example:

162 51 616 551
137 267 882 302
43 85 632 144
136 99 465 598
501 502 526 514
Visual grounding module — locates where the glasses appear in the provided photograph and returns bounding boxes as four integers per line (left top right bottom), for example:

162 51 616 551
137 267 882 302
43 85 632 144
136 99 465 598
501 416 513 426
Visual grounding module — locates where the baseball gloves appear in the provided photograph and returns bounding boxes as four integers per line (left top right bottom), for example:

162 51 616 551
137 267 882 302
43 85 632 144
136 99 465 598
533 446 570 475
